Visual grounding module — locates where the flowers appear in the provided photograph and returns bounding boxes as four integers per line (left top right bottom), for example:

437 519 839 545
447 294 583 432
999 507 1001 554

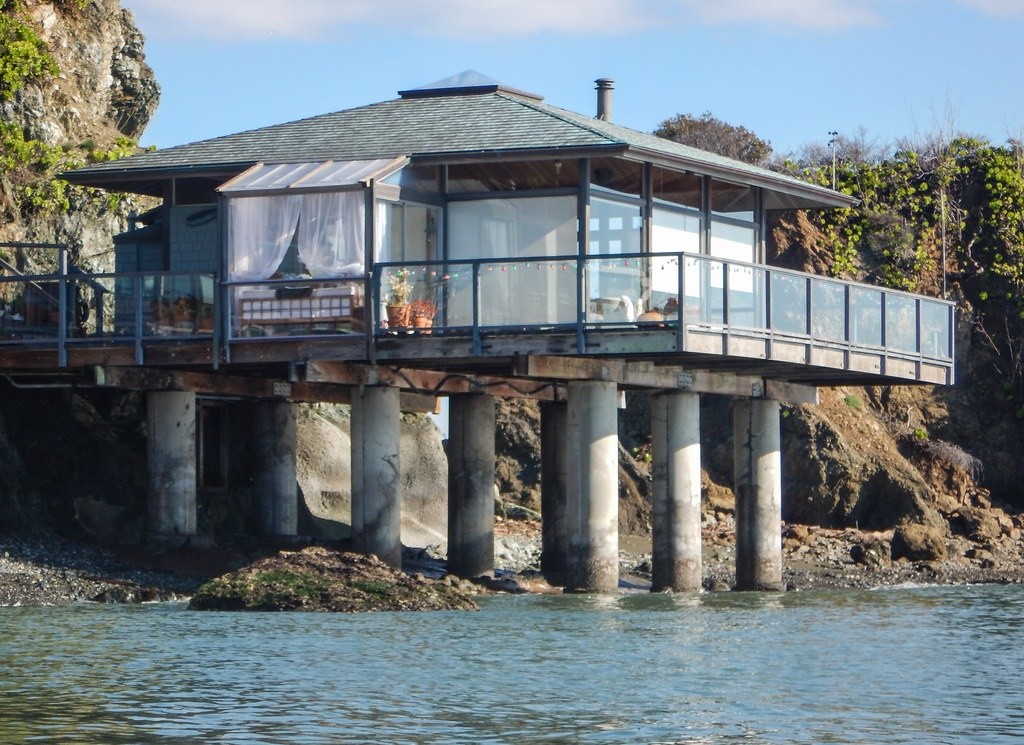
380 267 414 306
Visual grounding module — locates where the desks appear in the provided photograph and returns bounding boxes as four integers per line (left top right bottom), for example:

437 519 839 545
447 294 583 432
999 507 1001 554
592 298 625 315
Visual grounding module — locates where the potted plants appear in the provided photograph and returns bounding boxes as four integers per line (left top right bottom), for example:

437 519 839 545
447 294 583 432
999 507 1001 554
411 299 439 334
151 295 214 330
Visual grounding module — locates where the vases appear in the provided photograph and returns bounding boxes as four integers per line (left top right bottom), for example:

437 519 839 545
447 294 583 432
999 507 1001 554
386 305 411 327
